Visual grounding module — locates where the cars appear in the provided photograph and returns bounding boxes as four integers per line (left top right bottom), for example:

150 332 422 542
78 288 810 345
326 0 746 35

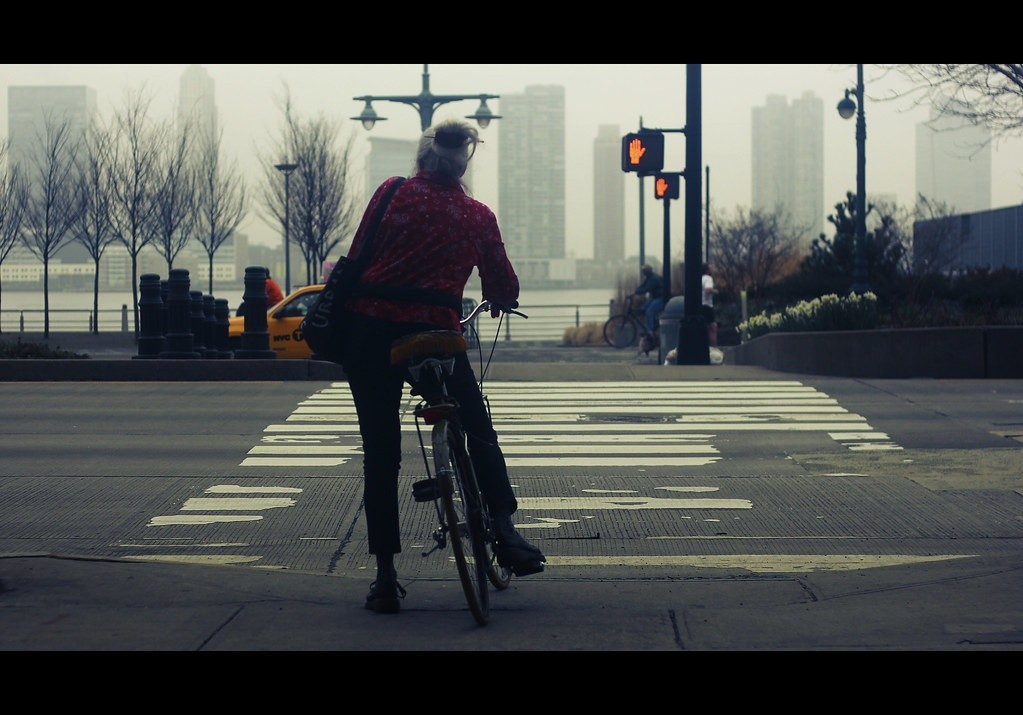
228 284 329 360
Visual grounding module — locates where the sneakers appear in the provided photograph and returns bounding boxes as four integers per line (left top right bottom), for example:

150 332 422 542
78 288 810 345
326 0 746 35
363 573 408 616
495 528 547 577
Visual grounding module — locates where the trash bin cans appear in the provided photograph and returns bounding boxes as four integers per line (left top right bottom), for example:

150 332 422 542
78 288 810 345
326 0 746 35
659 296 686 365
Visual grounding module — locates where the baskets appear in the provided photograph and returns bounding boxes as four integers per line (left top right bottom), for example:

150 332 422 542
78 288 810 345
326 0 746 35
460 298 480 349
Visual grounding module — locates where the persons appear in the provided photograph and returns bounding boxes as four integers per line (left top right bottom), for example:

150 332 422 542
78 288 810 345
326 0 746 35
341 121 546 617
702 263 718 347
265 267 284 307
634 264 662 336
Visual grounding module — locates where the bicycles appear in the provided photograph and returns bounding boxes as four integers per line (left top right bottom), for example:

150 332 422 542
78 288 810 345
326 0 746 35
391 298 529 625
603 293 650 350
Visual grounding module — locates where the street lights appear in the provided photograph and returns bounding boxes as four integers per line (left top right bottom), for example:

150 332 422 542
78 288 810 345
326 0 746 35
351 94 501 150
274 164 300 298
837 87 868 293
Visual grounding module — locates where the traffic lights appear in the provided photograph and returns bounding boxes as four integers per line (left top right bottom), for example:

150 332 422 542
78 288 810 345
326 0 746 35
654 173 679 199
622 133 664 173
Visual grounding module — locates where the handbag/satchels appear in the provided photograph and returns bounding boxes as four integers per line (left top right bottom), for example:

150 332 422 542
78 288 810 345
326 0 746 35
299 256 366 361
